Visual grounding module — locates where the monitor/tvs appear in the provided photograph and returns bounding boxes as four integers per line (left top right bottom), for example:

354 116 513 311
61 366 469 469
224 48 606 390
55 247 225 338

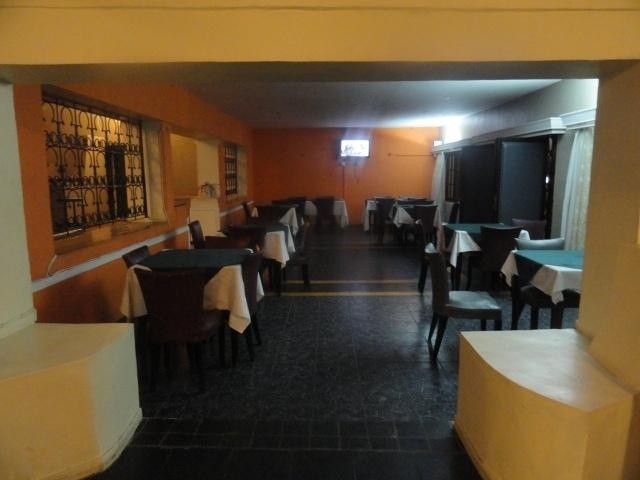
339 138 371 158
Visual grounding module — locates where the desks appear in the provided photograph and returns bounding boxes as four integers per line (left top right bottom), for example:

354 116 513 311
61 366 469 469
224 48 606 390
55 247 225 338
305 201 348 232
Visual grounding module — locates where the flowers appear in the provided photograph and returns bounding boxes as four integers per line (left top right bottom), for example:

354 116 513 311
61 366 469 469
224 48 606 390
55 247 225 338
198 182 215 198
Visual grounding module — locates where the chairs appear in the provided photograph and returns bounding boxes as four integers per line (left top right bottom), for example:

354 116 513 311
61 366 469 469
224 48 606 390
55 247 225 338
362 198 583 359
121 196 335 372
